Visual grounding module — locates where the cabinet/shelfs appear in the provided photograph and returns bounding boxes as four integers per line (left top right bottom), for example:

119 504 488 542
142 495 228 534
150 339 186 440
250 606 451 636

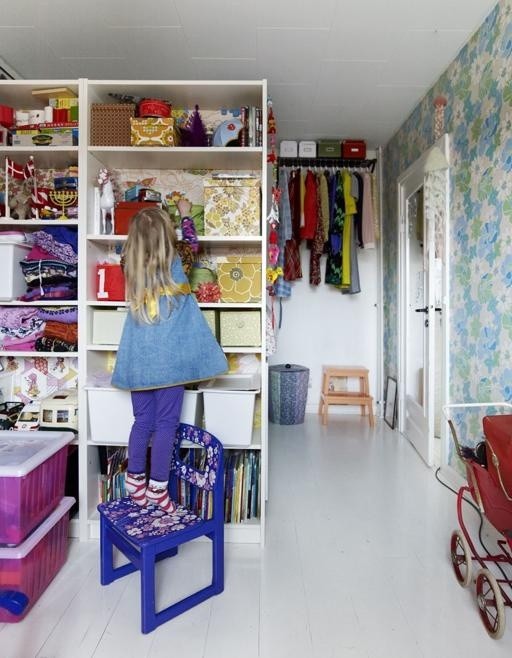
1 74 275 553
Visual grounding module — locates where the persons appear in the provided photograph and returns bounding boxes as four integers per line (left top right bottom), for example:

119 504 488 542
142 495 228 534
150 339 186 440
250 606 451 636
112 198 230 517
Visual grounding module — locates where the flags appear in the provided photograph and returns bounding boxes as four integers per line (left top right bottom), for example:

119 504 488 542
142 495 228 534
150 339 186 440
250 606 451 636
8 159 35 180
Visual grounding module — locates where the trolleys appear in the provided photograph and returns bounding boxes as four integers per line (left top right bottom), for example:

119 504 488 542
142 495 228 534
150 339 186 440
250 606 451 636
442 402 512 640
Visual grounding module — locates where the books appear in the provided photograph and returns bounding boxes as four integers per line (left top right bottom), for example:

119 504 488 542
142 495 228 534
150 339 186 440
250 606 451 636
32 87 79 105
97 449 261 526
241 105 263 147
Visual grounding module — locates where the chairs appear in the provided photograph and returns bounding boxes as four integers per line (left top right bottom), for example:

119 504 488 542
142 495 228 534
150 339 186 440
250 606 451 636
98 421 227 636
318 365 377 428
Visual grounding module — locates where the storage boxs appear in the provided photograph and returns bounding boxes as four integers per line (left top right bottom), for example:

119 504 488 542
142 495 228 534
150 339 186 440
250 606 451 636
202 177 261 237
1 426 78 628
279 137 367 159
0 229 34 308
91 99 189 151
83 375 261 447
93 256 264 349
